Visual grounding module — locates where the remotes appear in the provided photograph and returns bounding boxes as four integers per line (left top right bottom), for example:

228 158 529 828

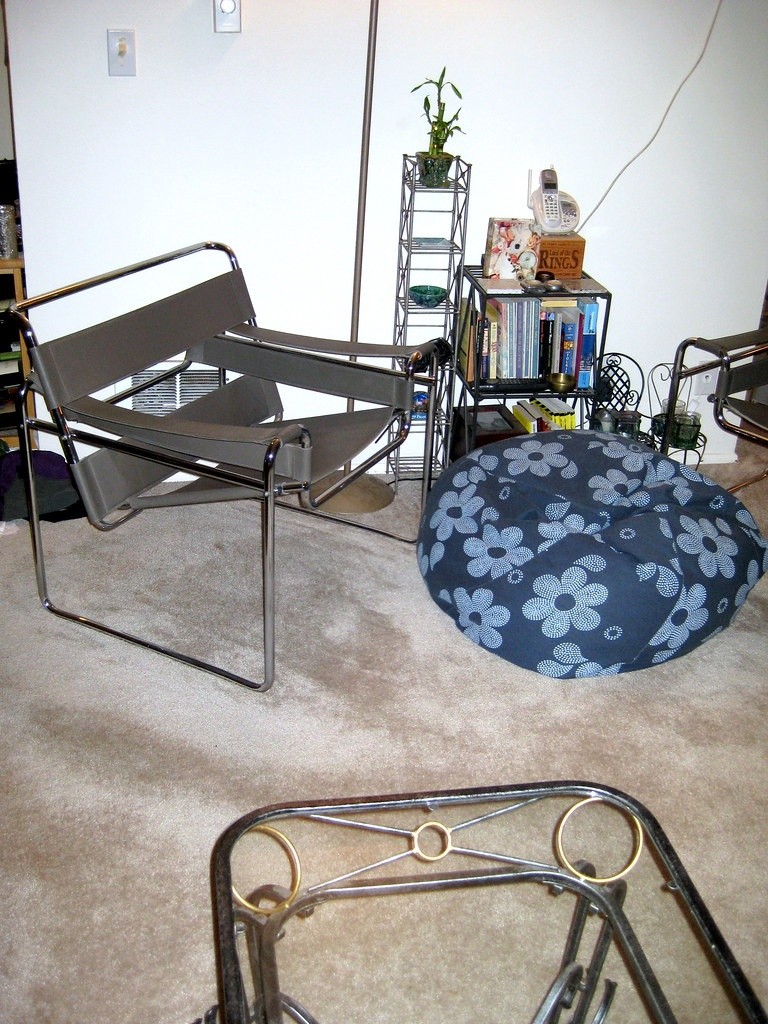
470 378 548 393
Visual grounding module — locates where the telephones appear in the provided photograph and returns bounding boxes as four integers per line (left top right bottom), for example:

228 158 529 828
527 164 581 236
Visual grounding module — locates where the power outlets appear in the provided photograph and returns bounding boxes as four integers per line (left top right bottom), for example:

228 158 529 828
693 361 718 396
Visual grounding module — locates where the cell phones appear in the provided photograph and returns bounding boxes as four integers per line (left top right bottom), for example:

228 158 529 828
520 279 546 294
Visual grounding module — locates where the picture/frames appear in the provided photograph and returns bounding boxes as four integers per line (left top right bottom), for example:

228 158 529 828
453 406 529 438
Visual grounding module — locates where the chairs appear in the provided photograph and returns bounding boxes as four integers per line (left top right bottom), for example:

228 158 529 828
661 327 768 496
6 241 439 693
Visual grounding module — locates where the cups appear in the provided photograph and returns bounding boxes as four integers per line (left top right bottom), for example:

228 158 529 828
686 411 701 420
0 205 17 259
674 415 701 449
662 398 686 415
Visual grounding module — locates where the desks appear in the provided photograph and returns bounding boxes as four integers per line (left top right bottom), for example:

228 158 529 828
209 783 768 1024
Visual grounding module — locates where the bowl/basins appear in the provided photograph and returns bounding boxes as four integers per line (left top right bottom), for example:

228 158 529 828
547 372 576 393
409 285 449 308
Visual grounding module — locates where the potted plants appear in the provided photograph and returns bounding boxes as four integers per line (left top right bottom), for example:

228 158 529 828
410 66 468 188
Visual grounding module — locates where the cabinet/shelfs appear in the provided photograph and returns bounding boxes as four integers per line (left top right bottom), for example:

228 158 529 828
0 253 38 449
440 264 612 469
386 153 474 479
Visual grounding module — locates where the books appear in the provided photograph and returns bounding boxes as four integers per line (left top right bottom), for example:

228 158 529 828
477 277 523 294
467 298 599 389
512 398 575 434
